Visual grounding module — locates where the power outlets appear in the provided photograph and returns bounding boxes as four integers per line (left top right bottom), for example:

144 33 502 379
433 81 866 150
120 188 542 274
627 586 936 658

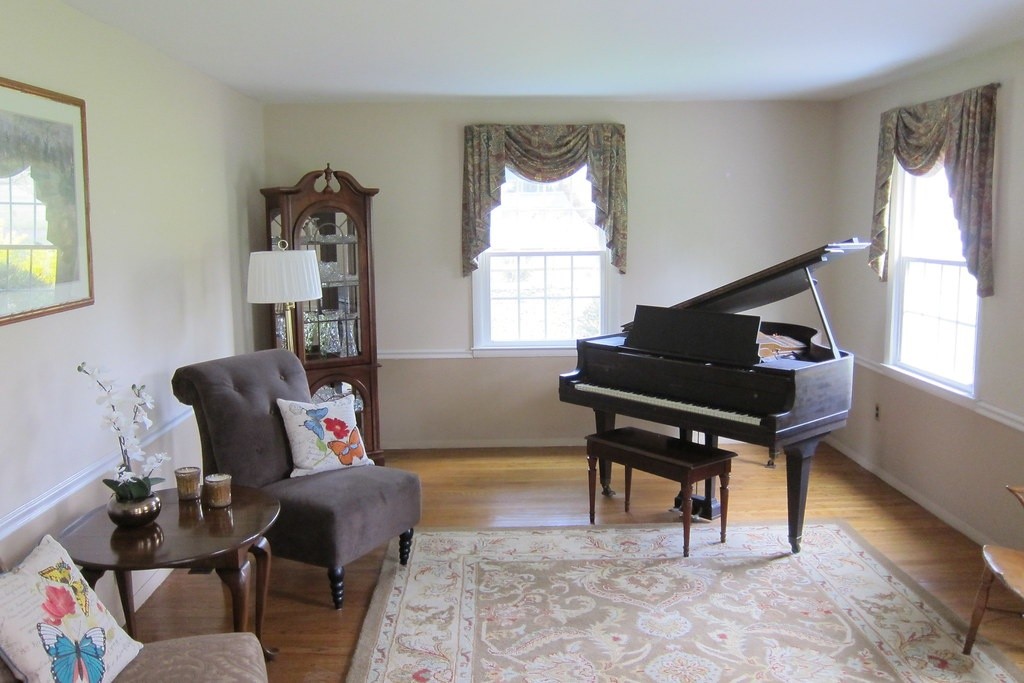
874 403 881 422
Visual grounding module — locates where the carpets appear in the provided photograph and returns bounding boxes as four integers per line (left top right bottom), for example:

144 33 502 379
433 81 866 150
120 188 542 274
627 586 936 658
342 519 1024 683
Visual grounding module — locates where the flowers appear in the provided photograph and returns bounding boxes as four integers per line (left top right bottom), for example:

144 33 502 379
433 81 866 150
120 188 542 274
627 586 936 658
75 357 171 501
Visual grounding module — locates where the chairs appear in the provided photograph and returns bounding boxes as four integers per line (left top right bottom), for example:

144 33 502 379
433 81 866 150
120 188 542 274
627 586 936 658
4 632 269 683
173 349 422 608
963 484 1024 656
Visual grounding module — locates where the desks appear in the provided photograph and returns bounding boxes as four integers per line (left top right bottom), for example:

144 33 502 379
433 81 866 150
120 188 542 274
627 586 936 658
59 483 283 660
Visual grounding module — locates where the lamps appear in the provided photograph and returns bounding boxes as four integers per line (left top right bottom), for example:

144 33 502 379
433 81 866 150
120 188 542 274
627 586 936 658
246 249 324 350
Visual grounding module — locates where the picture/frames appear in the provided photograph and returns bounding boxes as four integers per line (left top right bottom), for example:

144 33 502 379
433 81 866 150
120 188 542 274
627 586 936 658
0 76 96 328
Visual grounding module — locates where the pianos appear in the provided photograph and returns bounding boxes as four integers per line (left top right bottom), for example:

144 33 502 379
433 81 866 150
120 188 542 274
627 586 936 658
556 235 872 554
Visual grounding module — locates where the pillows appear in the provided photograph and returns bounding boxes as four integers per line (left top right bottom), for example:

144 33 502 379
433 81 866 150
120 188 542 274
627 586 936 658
0 534 145 683
275 393 376 479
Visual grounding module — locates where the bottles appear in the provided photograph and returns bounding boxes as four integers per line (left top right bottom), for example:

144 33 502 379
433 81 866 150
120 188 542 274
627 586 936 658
340 312 359 357
318 309 342 352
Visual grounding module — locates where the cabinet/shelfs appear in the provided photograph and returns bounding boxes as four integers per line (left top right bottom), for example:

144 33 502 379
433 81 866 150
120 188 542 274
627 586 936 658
262 162 386 467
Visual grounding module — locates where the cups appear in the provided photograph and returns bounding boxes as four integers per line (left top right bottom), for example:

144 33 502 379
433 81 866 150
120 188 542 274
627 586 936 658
205 473 232 508
174 466 201 500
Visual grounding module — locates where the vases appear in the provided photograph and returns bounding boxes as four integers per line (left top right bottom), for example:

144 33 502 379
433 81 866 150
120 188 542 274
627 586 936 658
110 522 164 558
107 491 163 527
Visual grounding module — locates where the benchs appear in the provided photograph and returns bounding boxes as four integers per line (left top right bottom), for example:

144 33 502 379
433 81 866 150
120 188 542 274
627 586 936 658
585 427 738 558
